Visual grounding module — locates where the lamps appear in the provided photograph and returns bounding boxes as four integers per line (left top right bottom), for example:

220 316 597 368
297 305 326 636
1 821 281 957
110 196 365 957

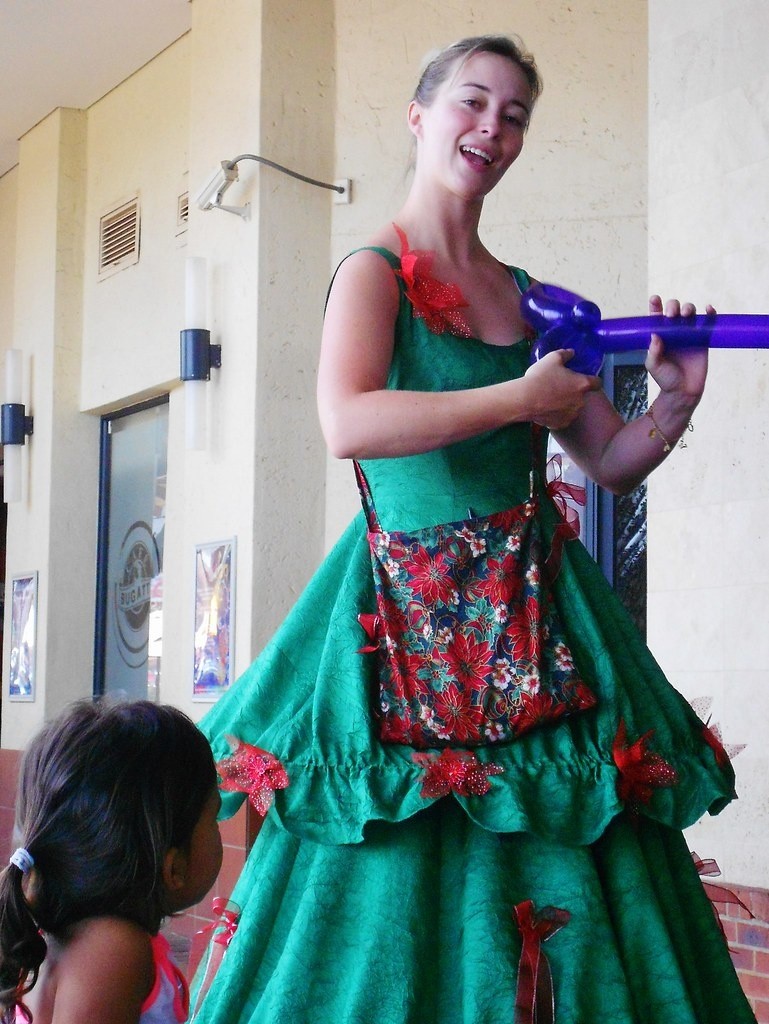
1 404 33 446
180 329 221 381
196 154 351 220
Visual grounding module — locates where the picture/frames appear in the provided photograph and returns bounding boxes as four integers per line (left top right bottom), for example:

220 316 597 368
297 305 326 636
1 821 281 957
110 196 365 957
8 570 37 702
192 536 237 703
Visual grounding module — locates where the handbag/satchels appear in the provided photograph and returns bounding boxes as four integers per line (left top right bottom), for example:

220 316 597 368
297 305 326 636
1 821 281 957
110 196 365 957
367 497 596 749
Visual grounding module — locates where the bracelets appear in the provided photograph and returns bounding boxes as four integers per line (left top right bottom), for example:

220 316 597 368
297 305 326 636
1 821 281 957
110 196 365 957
647 406 693 452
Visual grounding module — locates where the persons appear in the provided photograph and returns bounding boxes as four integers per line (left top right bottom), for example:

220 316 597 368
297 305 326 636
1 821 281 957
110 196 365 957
0 697 223 1024
174 38 758 1024
